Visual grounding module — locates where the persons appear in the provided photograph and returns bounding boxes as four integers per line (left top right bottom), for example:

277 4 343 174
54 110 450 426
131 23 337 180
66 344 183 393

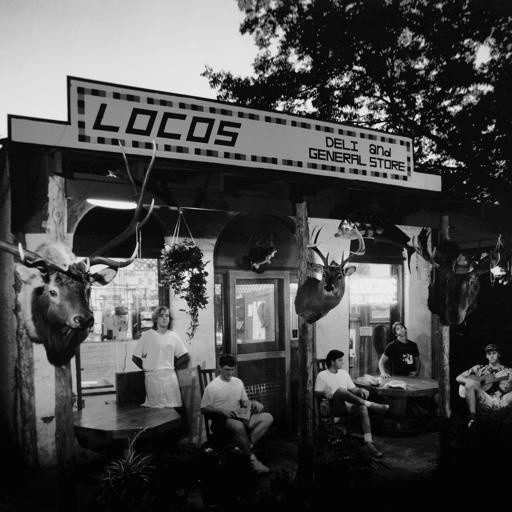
131 303 190 424
311 348 391 459
378 321 421 380
454 341 512 428
199 353 275 475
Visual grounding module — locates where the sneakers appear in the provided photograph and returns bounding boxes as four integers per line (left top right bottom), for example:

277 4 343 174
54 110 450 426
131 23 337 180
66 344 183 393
251 461 270 472
373 402 390 414
468 420 478 434
368 441 381 457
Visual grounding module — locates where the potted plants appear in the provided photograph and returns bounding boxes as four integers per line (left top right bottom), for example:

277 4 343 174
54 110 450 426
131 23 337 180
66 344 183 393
158 241 210 347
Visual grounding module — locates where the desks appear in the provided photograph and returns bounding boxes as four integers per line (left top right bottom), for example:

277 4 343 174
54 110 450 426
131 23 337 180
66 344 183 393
354 374 440 439
72 402 180 441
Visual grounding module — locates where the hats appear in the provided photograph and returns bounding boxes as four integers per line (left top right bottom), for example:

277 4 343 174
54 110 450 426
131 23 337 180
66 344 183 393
485 344 499 352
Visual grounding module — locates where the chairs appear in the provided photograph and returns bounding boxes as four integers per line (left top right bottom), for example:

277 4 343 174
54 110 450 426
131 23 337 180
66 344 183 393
197 365 222 453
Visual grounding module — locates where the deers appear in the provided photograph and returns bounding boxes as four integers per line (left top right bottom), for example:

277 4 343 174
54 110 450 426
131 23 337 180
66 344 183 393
0 139 157 367
412 225 502 326
295 225 366 324
